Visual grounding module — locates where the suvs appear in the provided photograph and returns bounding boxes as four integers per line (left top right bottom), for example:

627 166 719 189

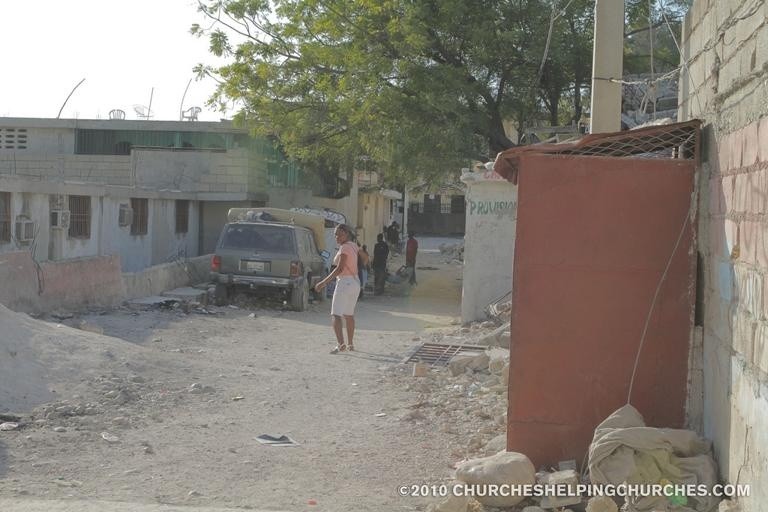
206 221 328 314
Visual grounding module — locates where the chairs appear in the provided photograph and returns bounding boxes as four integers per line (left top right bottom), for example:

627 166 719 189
108 108 127 120
132 103 154 120
180 105 202 122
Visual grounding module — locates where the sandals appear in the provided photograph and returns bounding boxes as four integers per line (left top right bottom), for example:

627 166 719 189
329 342 347 354
346 344 354 352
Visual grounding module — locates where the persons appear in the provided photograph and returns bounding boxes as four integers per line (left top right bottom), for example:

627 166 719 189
388 221 397 240
405 231 419 288
312 223 369 356
393 224 405 250
385 265 408 283
370 232 389 297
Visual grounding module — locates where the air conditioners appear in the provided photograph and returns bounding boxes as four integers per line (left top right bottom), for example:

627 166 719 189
12 219 38 243
48 208 72 231
117 204 134 229
